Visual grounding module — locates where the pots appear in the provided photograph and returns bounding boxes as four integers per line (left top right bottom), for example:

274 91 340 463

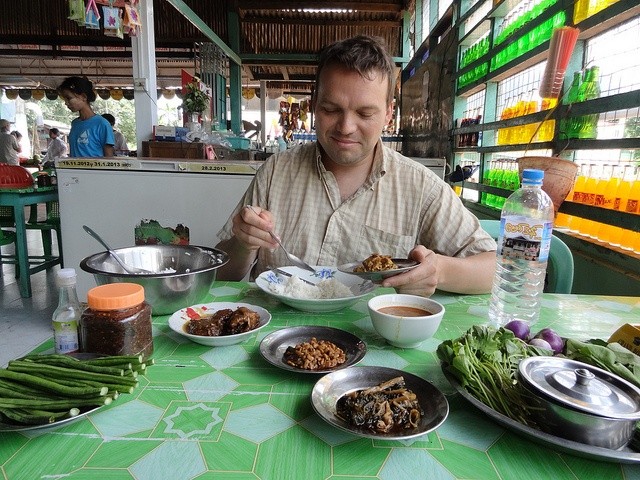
514 356 640 450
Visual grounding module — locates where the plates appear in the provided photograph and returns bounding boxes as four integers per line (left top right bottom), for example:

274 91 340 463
310 366 450 441
0 353 104 433
258 325 367 374
255 266 380 313
168 301 273 347
337 258 422 284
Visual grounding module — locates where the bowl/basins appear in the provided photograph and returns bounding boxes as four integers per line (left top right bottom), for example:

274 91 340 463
367 294 446 349
80 244 231 315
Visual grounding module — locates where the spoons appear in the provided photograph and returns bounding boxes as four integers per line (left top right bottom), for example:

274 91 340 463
246 205 315 271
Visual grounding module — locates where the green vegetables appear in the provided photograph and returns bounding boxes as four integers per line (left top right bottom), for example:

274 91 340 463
435 323 555 431
556 336 640 390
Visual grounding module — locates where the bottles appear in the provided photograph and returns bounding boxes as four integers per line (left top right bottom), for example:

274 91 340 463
578 65 601 139
37 171 51 188
51 268 85 355
211 116 219 140
620 165 640 251
568 68 591 139
591 163 611 237
490 0 566 73
598 164 622 241
458 107 483 147
50 163 57 186
608 164 634 246
578 164 599 234
487 169 554 332
458 34 490 89
497 88 558 146
561 71 583 139
381 130 403 152
569 164 589 230
265 127 317 154
81 283 153 362
555 182 574 227
453 158 521 210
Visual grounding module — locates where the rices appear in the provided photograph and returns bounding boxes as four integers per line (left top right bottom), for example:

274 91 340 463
135 266 176 275
267 273 354 300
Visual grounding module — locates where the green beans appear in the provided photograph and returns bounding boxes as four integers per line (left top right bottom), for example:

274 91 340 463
0 352 156 425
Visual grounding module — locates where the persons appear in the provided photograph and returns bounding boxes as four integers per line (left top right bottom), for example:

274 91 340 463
38 127 68 168
215 33 500 297
9 131 22 142
100 113 130 156
56 75 116 158
0 118 23 167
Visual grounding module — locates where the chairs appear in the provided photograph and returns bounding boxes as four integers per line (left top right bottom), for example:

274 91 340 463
475 219 576 295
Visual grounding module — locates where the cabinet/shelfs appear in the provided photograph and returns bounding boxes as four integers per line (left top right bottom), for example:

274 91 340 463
402 1 639 271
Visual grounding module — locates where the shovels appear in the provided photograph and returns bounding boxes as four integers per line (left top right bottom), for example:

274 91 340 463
82 222 156 277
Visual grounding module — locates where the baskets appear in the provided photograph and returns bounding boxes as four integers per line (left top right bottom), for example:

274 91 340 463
1 163 34 187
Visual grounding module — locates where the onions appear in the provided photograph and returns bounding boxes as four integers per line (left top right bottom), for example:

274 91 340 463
533 328 562 353
505 319 530 339
529 338 550 350
